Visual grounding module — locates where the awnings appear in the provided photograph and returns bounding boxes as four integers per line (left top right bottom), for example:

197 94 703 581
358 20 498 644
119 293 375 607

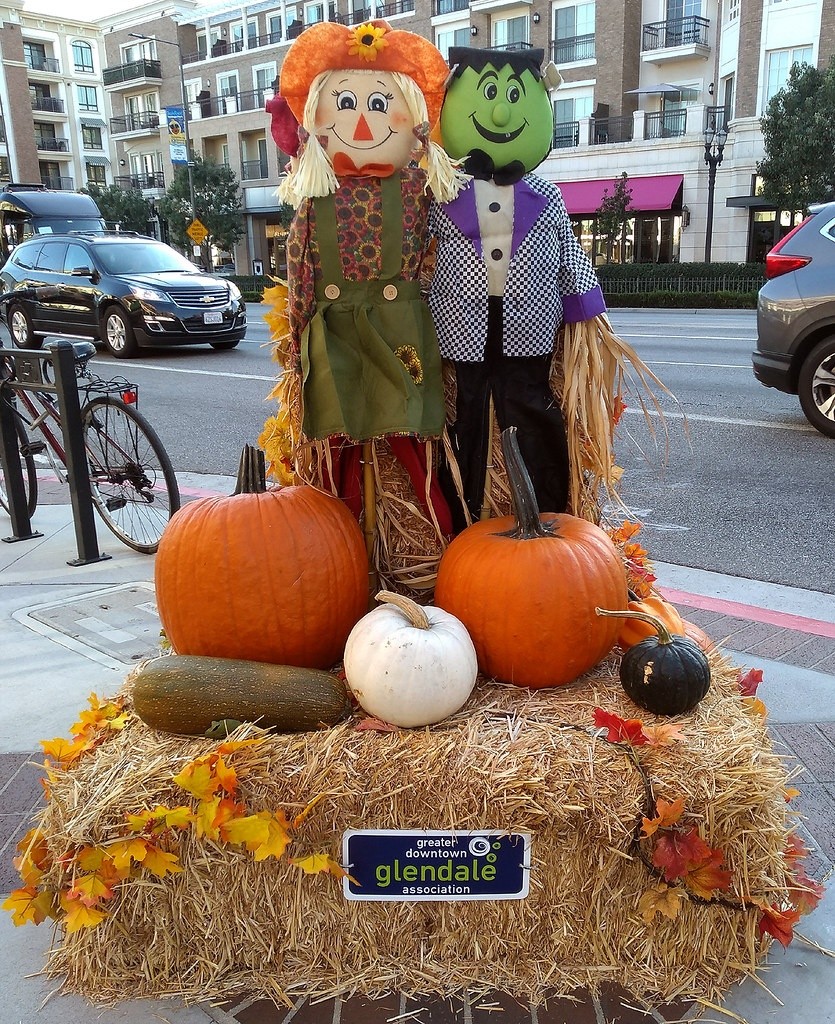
79 117 107 134
84 156 112 171
552 174 684 214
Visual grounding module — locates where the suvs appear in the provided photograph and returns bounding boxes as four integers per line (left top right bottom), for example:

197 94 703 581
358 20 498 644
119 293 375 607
749 201 835 440
0 228 247 359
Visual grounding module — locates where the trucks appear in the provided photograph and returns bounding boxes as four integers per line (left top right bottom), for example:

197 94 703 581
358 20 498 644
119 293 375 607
0 182 111 268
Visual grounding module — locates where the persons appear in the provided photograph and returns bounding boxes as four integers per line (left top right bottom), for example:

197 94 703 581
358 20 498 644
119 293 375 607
422 46 609 535
274 15 455 545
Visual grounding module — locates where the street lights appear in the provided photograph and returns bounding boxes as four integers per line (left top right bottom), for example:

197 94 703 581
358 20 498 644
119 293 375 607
127 33 201 270
701 127 729 263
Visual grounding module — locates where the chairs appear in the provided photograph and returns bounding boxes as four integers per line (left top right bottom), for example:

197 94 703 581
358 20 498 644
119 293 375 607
130 179 140 189
144 177 156 188
597 130 616 144
661 128 672 138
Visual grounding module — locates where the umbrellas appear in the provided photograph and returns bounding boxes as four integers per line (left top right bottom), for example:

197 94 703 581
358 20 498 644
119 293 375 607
624 81 701 136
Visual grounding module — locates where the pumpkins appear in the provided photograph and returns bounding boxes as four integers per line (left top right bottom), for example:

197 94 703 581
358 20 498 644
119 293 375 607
344 590 479 729
618 589 685 653
594 607 710 717
133 656 352 735
681 618 719 657
156 444 370 670
435 426 629 690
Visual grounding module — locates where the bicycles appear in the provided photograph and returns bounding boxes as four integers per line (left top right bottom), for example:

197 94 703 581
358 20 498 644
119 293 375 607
0 284 179 557
